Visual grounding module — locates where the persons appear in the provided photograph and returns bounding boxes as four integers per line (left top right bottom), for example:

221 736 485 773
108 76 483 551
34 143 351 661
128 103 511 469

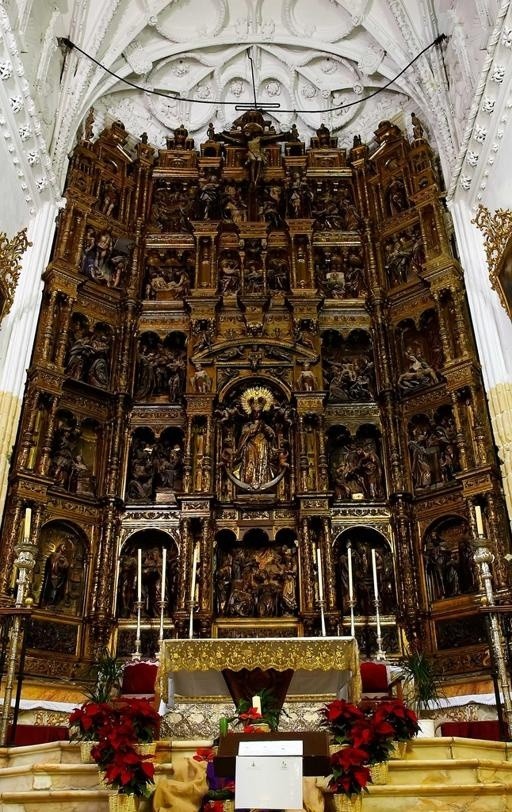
40 105 478 621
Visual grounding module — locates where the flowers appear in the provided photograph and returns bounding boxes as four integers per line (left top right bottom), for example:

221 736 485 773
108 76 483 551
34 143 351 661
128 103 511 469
315 695 424 802
68 692 163 801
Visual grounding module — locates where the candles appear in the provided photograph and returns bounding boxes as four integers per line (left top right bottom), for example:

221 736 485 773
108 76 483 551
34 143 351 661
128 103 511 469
23 507 34 538
474 504 485 537
135 548 144 603
347 548 354 603
159 548 169 604
315 547 327 601
370 548 379 601
190 548 200 600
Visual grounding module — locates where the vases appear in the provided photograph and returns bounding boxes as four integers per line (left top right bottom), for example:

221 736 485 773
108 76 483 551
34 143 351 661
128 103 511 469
106 793 137 811
333 793 363 812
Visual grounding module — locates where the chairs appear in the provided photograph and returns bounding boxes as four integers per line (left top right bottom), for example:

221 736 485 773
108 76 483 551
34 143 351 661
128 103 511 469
359 660 404 702
111 659 159 699
